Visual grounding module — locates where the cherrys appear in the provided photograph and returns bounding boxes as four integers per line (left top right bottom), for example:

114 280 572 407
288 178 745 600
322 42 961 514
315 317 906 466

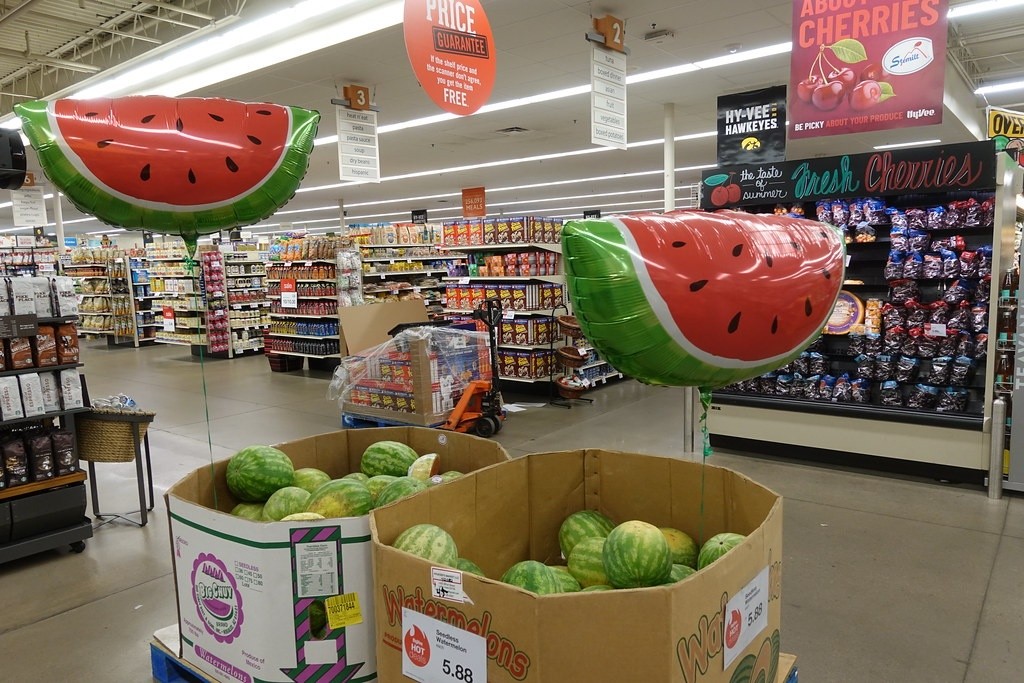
797 44 883 112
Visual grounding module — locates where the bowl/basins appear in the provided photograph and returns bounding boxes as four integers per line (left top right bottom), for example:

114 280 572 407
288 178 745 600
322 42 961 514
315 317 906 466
557 375 585 398
557 346 589 368
557 315 583 338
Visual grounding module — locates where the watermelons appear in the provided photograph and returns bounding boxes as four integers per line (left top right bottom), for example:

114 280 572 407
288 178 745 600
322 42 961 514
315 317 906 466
226 440 467 522
385 508 751 594
561 207 848 392
13 95 322 237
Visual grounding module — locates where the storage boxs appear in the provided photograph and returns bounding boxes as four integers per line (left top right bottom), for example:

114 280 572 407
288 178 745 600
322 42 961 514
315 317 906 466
344 216 567 415
134 227 207 345
147 425 800 683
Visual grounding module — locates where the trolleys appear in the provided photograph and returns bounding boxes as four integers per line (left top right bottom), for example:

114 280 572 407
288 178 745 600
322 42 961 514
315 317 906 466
339 297 509 439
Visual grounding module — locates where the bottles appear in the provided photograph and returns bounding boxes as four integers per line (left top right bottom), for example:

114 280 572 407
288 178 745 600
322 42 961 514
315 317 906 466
270 301 338 315
266 264 334 279
268 283 335 296
995 267 1020 431
271 340 339 355
271 320 338 336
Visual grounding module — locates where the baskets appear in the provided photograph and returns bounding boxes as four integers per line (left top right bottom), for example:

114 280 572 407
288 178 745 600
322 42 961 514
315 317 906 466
557 346 589 367
557 376 587 398
559 315 583 337
76 406 157 464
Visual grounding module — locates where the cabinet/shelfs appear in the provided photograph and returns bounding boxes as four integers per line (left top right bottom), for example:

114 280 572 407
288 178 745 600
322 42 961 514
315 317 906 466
222 250 273 359
708 150 1024 488
566 302 628 386
359 242 448 316
64 264 115 346
260 260 344 359
0 315 94 567
145 258 211 359
440 242 568 386
125 255 155 348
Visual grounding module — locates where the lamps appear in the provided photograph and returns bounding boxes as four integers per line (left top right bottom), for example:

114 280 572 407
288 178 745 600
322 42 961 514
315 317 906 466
0 0 404 141
973 75 1024 96
946 0 1024 19
